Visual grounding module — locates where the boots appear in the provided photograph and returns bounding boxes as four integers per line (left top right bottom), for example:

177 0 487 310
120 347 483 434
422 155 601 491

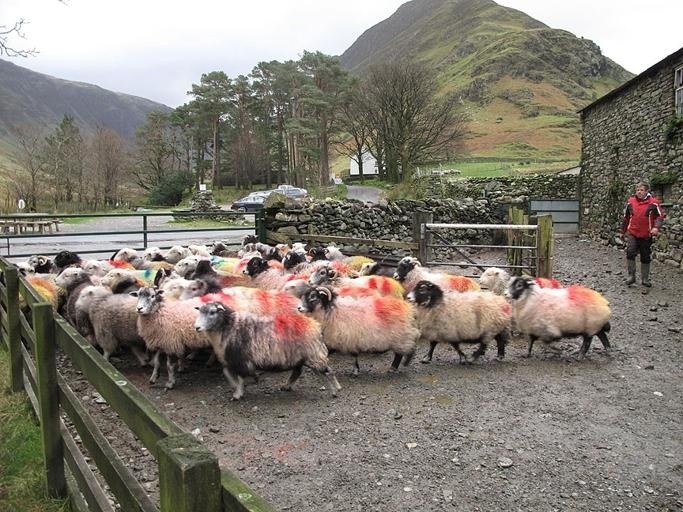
625 259 636 285
640 263 652 287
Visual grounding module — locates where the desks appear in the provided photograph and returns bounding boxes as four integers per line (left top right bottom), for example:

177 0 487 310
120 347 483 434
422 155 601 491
0 212 48 234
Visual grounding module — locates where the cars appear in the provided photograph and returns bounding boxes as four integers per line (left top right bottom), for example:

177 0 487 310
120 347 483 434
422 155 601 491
231 185 307 213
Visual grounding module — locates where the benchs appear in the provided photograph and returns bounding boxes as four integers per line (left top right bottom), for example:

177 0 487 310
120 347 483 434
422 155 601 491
0 220 63 236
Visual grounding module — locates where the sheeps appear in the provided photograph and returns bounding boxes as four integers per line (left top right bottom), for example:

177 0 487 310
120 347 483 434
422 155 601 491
1 234 613 401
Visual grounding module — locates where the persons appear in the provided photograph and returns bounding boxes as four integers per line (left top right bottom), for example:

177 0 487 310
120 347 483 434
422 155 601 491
615 183 666 287
